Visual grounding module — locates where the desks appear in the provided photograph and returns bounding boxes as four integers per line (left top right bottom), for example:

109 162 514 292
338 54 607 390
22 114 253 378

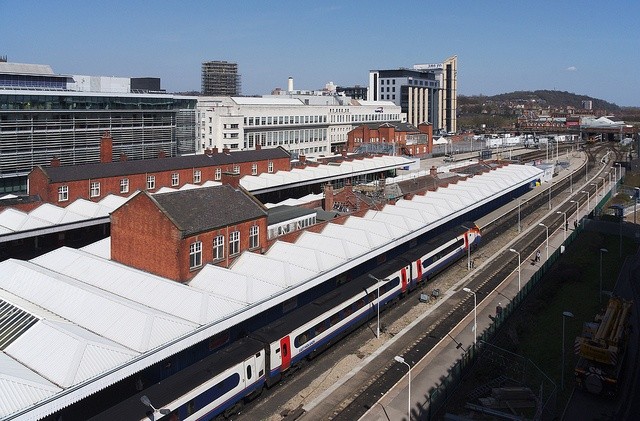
600 248 608 304
619 216 626 257
570 201 578 227
509 249 520 291
634 186 639 224
395 355 410 421
610 167 616 194
599 177 605 196
582 191 589 214
557 211 566 240
605 172 611 190
616 163 621 179
539 223 548 260
463 288 477 351
590 184 597 206
561 312 574 391
513 197 528 233
621 125 633 144
368 274 390 339
461 225 471 270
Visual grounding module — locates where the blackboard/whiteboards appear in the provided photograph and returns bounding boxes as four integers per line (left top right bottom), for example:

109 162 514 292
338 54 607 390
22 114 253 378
574 292 634 395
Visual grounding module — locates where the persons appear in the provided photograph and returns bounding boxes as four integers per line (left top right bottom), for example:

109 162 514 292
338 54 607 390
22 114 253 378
566 219 569 232
574 219 577 229
535 250 540 262
